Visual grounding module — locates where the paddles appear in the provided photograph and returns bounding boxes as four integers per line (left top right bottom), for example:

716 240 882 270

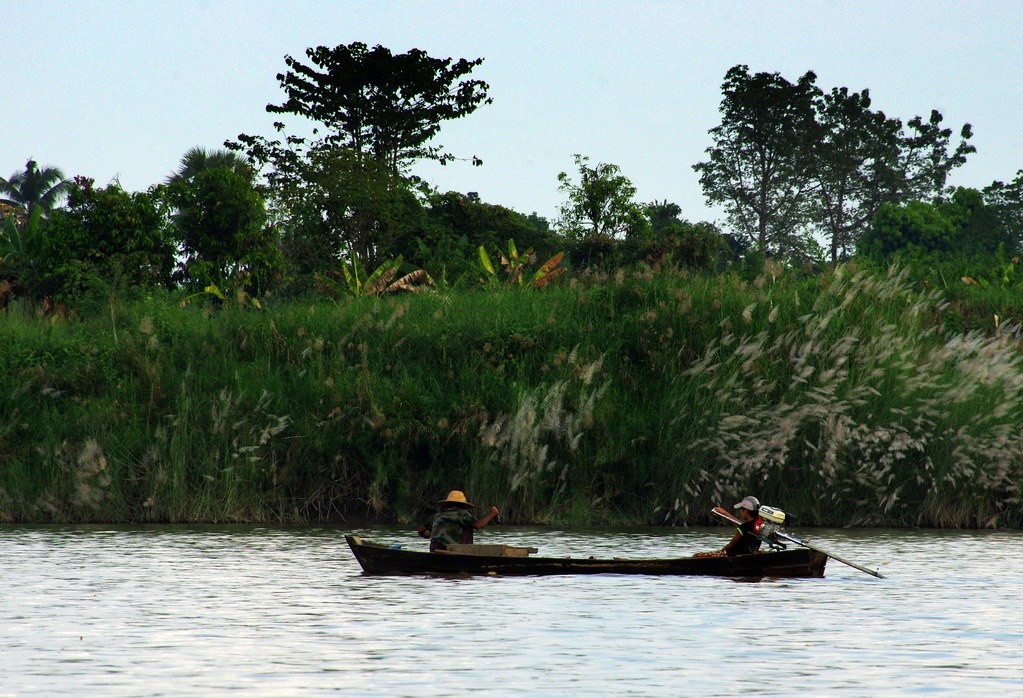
713 506 889 580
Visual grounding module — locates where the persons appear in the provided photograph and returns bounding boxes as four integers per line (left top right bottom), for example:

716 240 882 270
418 490 499 552
692 496 762 558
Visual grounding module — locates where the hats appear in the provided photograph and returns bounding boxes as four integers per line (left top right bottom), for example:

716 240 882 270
437 491 475 508
733 497 760 510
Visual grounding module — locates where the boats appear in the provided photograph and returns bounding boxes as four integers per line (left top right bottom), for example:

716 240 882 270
345 533 830 579
